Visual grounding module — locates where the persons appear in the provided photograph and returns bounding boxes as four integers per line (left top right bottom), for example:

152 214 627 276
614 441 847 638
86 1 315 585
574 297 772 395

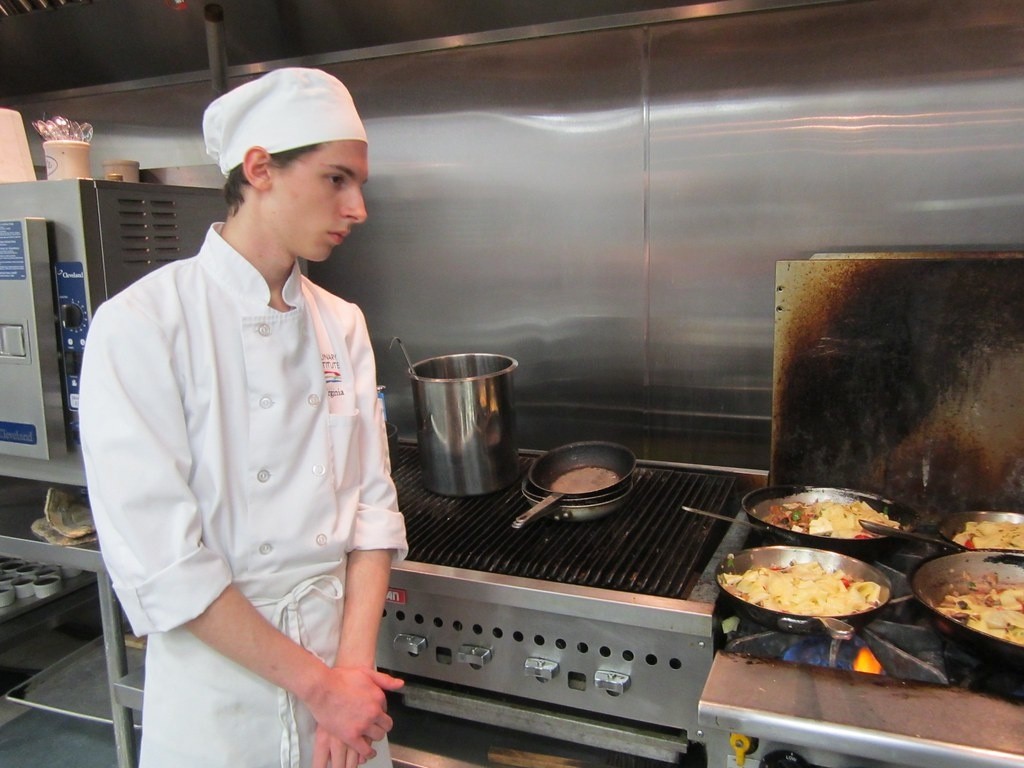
79 67 409 768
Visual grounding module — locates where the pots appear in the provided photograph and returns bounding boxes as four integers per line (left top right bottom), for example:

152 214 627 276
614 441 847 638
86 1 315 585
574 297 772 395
910 552 1024 647
859 511 1024 552
681 484 920 560
408 352 519 496
714 545 893 639
512 441 636 529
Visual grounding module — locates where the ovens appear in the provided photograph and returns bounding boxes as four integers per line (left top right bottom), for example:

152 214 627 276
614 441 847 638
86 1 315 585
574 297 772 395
0 180 222 488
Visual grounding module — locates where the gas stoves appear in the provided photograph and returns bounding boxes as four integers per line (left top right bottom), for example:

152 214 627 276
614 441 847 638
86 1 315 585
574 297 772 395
697 533 1024 768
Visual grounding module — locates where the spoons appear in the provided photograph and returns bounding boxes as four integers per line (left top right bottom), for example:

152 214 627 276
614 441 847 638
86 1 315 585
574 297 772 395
32 116 93 143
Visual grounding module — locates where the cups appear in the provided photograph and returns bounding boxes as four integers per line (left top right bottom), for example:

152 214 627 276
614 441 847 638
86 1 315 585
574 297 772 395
0 556 83 607
102 161 139 183
43 140 92 180
0 108 37 183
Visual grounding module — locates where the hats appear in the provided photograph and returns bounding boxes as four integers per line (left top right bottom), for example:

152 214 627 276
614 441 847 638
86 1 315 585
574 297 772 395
201 67 368 179
